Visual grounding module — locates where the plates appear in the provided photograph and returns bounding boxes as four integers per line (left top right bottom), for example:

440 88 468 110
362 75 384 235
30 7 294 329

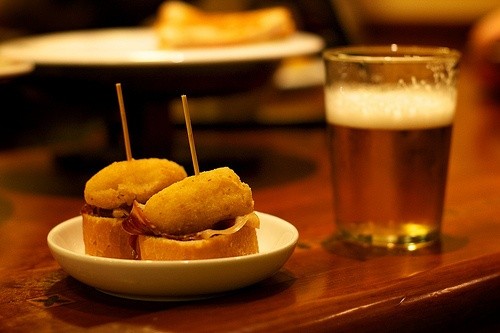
6 25 326 92
45 210 300 302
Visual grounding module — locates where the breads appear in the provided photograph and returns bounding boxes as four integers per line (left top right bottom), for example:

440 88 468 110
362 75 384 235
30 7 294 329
153 0 293 48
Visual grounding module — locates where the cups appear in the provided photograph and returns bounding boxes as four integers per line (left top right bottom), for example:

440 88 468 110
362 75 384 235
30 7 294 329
322 46 462 250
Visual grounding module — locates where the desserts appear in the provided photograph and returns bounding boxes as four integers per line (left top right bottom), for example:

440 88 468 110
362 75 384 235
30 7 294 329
120 167 260 261
79 158 187 260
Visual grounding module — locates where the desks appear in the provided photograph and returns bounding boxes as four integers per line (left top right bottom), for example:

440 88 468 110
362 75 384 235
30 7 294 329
0 70 500 333
